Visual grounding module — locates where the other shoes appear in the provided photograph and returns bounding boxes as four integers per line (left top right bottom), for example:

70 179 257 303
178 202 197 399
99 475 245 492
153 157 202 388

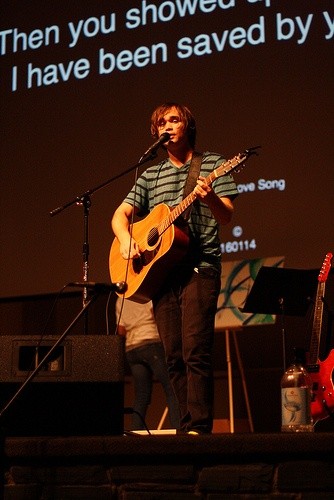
186 430 203 435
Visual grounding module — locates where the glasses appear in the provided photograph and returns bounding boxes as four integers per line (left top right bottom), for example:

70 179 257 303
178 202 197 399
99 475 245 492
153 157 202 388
157 118 184 130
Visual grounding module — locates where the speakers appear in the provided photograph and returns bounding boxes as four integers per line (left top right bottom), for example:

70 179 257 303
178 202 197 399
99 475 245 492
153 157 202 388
0 382 125 439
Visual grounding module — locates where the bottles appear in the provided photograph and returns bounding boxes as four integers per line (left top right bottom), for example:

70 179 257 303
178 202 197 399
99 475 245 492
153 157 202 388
279 345 309 434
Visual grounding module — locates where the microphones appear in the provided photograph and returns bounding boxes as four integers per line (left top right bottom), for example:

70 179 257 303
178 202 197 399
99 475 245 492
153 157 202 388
67 281 128 294
144 133 170 158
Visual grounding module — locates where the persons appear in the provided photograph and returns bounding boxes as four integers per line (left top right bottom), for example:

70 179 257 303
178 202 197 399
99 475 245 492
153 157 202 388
111 101 239 435
116 297 180 430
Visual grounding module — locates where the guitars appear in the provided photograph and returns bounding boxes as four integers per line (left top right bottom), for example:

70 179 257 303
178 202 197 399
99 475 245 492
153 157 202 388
302 254 334 421
108 152 248 305
